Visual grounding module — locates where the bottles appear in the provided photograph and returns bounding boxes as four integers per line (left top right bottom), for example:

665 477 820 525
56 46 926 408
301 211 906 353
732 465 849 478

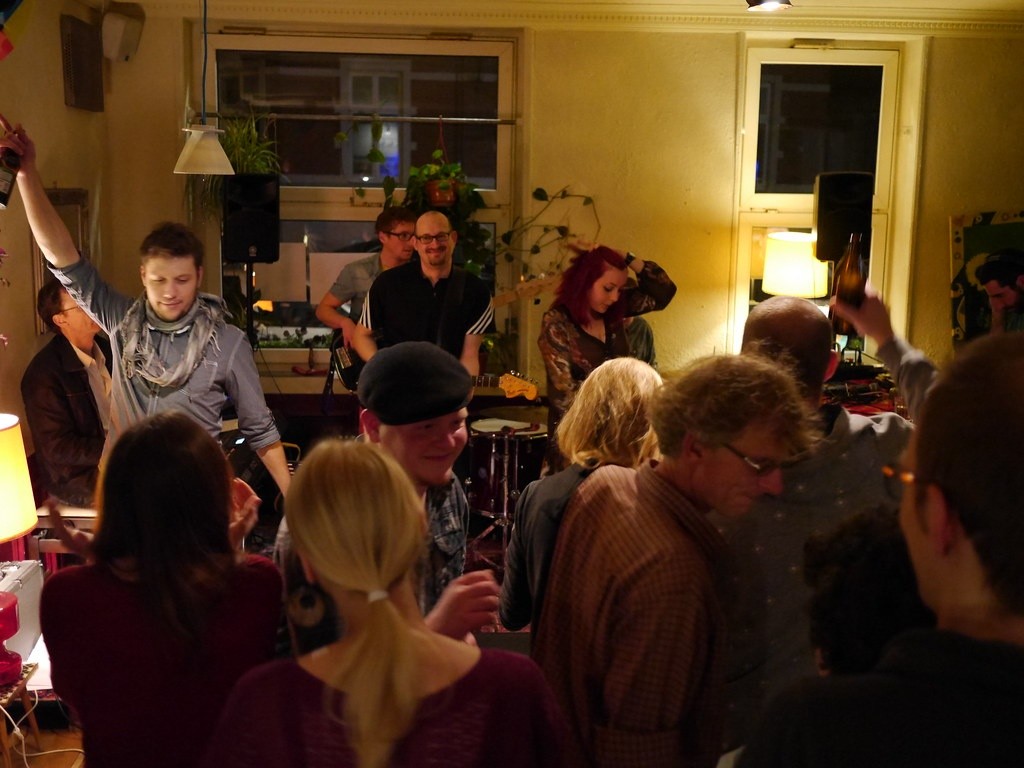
0 130 21 210
827 232 866 337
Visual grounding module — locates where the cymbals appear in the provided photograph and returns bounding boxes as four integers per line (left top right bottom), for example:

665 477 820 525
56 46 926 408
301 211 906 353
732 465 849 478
479 404 549 425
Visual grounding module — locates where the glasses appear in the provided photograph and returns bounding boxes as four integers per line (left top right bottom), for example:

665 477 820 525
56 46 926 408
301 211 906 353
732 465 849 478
724 443 781 476
386 232 415 242
418 232 451 244
63 305 78 313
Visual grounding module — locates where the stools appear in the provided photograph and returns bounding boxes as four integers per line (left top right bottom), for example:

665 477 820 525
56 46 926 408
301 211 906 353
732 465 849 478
470 416 551 545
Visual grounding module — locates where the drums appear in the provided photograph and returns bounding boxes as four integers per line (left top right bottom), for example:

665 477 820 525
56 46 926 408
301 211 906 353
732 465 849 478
469 416 548 520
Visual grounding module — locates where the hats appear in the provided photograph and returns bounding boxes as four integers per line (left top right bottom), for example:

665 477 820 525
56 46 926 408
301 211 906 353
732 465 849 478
358 341 476 425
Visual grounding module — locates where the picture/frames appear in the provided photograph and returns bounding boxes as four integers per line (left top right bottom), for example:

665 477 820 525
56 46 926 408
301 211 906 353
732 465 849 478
30 187 93 335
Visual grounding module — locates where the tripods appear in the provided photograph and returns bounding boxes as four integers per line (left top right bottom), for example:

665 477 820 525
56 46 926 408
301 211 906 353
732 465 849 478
463 435 513 571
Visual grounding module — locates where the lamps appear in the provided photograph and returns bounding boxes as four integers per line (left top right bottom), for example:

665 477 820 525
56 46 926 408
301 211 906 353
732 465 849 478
762 231 828 299
746 0 794 12
173 0 235 175
0 412 39 686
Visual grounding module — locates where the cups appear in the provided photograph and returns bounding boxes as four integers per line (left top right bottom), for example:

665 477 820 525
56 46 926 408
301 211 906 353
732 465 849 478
894 394 913 423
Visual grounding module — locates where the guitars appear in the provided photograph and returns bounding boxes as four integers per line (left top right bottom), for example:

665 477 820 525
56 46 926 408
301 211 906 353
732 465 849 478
468 367 540 402
331 272 554 396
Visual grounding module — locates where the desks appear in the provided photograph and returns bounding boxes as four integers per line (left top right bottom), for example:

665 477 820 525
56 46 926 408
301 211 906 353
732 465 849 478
0 660 45 768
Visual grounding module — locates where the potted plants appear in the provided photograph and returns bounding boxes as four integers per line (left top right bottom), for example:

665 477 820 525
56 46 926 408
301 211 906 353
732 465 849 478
399 151 490 281
179 100 291 227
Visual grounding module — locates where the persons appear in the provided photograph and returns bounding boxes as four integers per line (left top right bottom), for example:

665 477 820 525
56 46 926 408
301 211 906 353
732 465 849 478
0 110 291 509
38 412 288 768
315 206 497 379
201 437 586 767
975 248 1024 335
716 331 1024 767
502 283 946 767
272 342 502 657
537 237 678 477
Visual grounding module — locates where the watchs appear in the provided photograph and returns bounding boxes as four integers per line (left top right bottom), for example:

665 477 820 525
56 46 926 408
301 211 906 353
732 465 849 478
625 252 636 265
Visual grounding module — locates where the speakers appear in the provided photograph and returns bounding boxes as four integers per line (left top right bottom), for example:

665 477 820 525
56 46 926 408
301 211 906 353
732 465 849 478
222 174 279 265
101 15 141 62
812 172 874 262
